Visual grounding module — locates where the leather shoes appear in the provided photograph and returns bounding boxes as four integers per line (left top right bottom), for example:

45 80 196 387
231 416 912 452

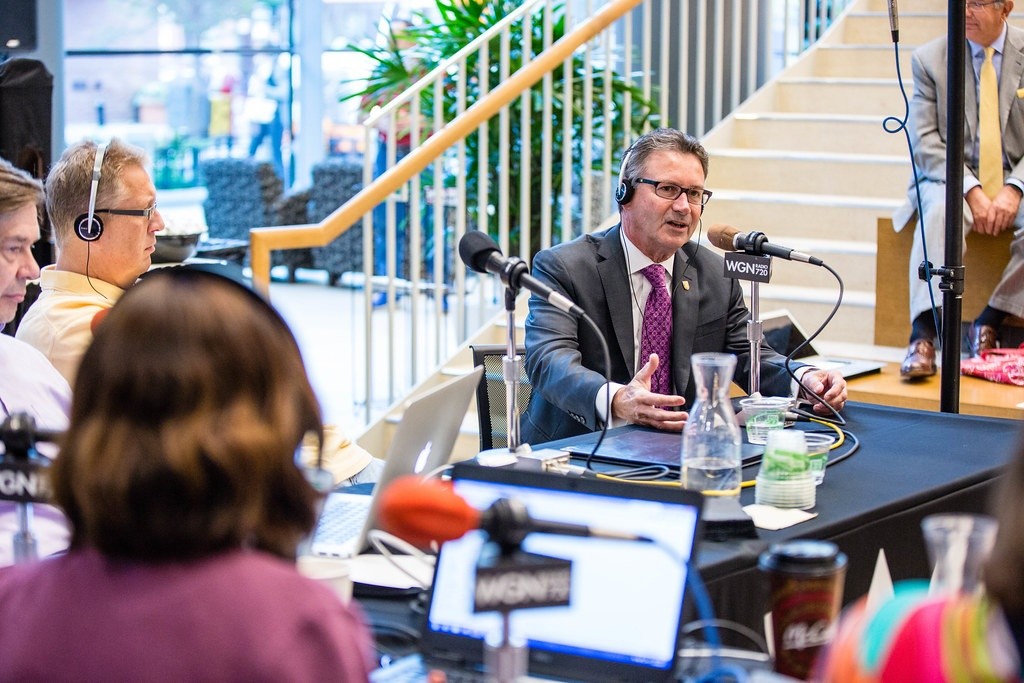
900 338 938 378
965 318 999 358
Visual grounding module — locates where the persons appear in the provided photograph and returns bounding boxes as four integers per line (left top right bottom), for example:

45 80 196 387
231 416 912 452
519 127 849 446
899 0 1024 377
202 44 287 160
14 134 388 492
0 154 77 568
804 418 1023 683
0 264 379 683
356 18 451 315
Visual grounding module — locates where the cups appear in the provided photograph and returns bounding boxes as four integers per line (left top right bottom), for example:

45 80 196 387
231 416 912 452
740 397 835 511
758 540 847 682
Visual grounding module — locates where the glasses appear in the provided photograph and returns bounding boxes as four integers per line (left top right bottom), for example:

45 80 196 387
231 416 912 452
630 177 713 205
94 201 157 220
966 1 997 12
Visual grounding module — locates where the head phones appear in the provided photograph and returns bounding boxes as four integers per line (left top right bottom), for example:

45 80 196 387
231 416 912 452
614 135 705 217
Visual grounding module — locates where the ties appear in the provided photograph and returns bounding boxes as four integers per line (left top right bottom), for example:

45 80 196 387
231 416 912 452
978 47 1003 202
639 263 675 411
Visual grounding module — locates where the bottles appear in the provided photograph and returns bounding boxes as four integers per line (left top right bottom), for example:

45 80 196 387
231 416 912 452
682 352 743 503
925 515 997 600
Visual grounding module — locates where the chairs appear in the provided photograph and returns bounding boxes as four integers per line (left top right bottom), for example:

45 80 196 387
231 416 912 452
202 159 314 281
301 158 412 291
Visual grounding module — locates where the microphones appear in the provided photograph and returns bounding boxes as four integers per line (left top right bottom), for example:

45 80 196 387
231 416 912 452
707 224 823 266
888 1 900 41
375 474 651 548
458 230 588 318
74 142 109 241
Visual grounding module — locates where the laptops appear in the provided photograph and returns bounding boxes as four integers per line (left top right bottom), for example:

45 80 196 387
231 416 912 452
367 461 707 683
758 308 887 379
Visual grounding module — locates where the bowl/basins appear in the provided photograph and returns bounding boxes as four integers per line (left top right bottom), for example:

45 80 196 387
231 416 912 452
151 223 209 264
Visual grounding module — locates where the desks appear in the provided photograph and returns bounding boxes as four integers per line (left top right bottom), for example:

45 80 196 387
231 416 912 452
324 395 1024 682
